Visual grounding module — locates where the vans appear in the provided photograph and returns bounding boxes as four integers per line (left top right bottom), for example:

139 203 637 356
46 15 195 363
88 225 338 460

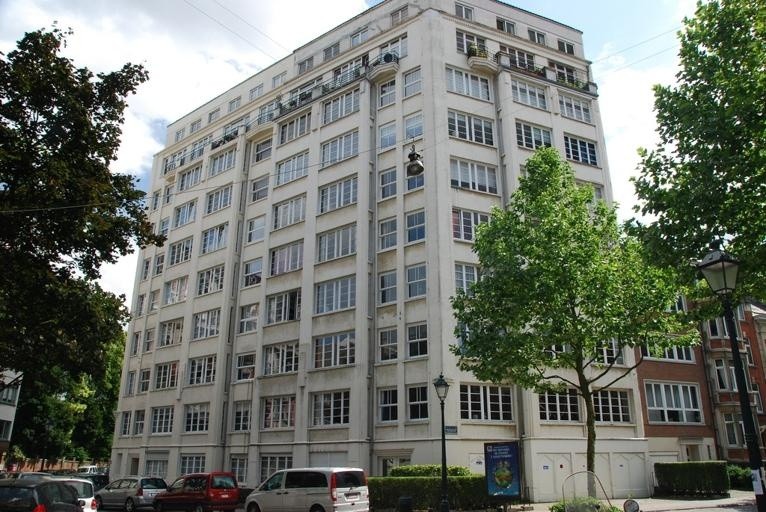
151 471 242 512
245 467 370 512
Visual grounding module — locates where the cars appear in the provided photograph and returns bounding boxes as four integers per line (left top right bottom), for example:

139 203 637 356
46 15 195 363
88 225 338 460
0 465 106 512
93 476 169 512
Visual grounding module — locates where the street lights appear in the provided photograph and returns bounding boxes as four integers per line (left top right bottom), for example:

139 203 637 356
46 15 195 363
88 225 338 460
432 372 450 512
696 240 766 512
